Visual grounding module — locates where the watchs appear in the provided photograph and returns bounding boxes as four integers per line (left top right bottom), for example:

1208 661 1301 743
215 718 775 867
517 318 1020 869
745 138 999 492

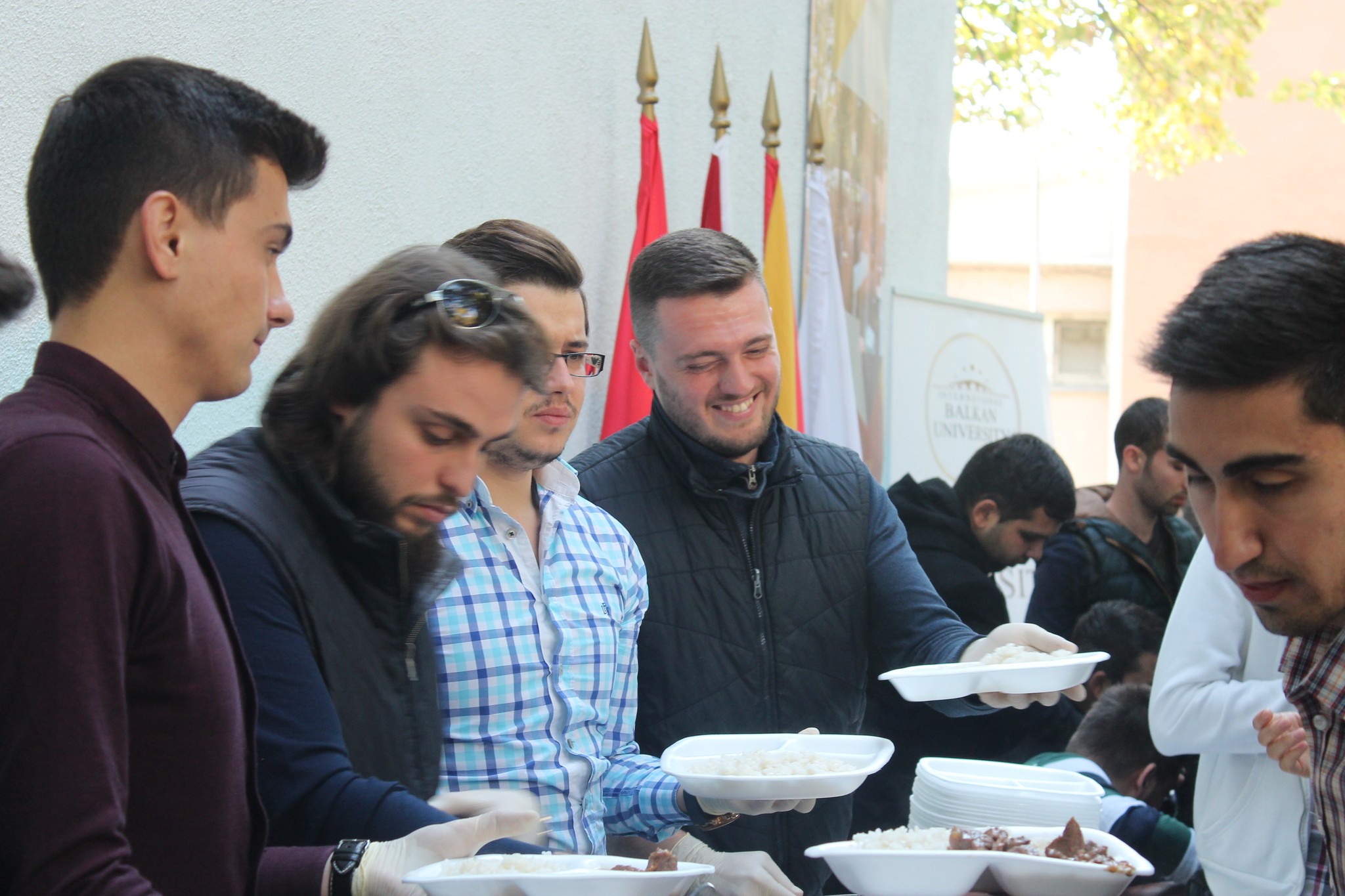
680 787 741 832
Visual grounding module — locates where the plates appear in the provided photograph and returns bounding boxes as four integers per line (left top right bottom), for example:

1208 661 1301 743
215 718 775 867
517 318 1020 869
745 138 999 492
399 652 1155 895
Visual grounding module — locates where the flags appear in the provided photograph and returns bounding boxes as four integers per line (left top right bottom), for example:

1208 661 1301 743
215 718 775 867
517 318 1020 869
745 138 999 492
761 149 806 434
697 133 731 233
797 162 864 461
602 112 670 442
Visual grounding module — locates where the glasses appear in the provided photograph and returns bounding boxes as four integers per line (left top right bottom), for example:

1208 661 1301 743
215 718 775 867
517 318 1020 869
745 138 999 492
545 351 606 377
394 278 528 330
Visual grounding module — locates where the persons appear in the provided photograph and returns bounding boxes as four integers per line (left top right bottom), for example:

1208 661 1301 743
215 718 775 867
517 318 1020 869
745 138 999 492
568 226 1089 896
1027 396 1204 639
0 51 541 896
181 239 552 852
1019 683 1211 896
434 217 820 855
1139 228 1345 896
1149 535 1309 896
1018 598 1169 767
851 430 1078 839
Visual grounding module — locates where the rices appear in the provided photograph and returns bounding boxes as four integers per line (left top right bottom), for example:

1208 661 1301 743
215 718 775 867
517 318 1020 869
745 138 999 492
979 641 1071 673
435 847 599 891
840 820 962 856
687 734 855 786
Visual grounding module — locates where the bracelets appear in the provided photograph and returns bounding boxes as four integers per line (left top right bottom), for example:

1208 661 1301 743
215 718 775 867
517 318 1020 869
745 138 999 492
328 836 372 896
